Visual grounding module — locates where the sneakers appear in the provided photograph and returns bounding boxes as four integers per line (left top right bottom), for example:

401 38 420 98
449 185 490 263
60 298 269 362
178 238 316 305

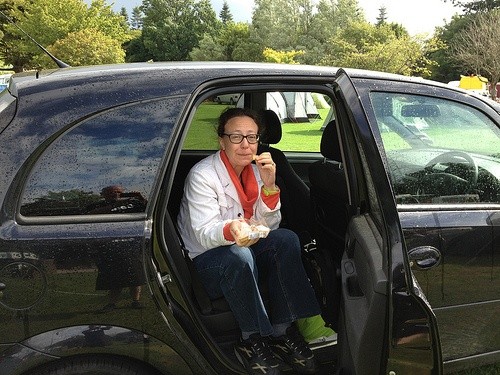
269 333 319 373
240 332 280 375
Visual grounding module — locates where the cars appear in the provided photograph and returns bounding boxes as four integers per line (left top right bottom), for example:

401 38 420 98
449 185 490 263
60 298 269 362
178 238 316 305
0 60 500 375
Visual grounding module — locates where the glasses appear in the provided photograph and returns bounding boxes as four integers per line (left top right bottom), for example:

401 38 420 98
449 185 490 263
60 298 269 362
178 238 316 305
221 132 260 144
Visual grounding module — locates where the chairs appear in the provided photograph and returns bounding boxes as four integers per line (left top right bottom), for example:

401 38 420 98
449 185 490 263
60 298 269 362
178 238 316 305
252 110 310 230
310 119 356 240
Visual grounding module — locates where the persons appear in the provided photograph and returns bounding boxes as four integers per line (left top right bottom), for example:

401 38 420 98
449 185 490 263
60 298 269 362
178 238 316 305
175 107 344 375
81 185 150 310
